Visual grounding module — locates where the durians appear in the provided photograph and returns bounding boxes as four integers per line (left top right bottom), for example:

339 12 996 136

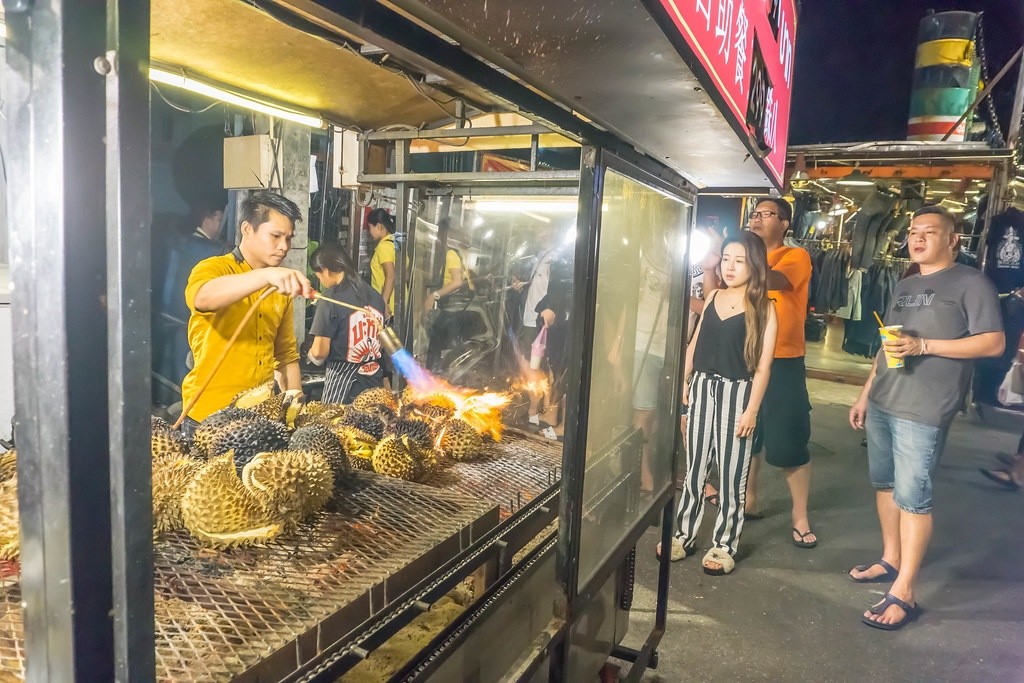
152 379 500 549
0 448 19 565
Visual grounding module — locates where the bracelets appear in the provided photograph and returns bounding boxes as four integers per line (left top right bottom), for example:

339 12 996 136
920 337 925 356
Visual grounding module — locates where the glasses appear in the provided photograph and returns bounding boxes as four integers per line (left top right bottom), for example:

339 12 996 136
750 211 780 219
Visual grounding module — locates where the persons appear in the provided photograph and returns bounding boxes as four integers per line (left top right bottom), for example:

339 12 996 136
305 243 387 402
656 231 777 575
182 191 312 441
158 195 232 407
367 205 723 510
980 431 1024 490
703 197 820 546
848 204 1006 631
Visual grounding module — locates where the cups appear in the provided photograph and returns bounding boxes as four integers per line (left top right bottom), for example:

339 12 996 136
530 343 546 370
878 324 905 369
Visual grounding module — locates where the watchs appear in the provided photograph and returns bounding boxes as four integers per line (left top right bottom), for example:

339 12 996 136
434 291 440 300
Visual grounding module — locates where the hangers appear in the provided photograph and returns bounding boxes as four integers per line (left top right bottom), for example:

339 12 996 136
793 237 852 251
878 255 913 269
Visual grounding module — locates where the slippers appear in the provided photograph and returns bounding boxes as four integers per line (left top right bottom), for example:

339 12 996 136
535 425 564 441
980 467 1024 489
793 528 817 548
861 593 918 629
997 451 1022 465
746 511 763 519
702 547 742 574
704 485 717 504
847 559 899 582
526 413 556 427
639 488 653 503
657 537 696 561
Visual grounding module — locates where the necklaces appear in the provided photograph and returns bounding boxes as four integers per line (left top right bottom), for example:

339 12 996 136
723 289 743 309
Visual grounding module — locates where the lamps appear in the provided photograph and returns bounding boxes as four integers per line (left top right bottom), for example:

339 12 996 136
149 57 329 133
828 199 849 216
943 193 968 206
835 161 875 185
460 195 610 214
790 155 812 182
